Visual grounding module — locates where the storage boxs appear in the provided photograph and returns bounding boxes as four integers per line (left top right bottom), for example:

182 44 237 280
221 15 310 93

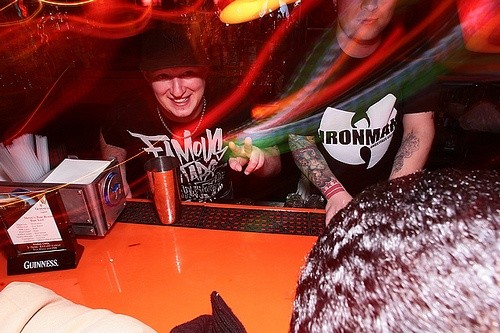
33 155 127 238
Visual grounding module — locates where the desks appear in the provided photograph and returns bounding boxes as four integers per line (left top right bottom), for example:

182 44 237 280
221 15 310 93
0 197 326 333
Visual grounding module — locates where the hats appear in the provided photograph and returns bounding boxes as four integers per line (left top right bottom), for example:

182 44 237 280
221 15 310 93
137 30 212 70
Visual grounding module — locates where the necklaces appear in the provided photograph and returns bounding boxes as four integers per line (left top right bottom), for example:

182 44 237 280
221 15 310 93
156 95 206 139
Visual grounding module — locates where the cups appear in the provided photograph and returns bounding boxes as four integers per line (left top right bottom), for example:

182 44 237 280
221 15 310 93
145 157 182 225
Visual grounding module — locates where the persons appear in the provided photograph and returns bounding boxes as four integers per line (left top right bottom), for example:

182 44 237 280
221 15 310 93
99 35 281 203
281 1 439 228
